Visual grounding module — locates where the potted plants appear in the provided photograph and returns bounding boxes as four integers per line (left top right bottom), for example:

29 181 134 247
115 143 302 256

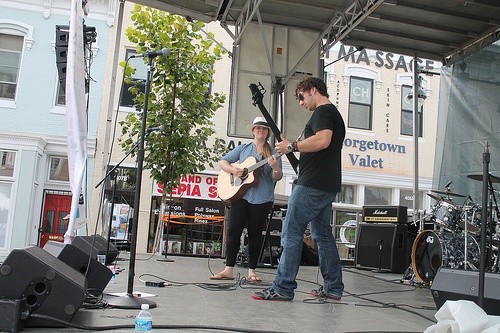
211 240 222 256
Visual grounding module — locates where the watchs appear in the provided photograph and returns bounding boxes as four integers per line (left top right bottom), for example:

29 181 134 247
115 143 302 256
286 143 293 152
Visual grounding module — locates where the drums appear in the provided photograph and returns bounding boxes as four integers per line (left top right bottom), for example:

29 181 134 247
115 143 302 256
459 202 491 233
410 230 481 287
431 201 463 232
486 220 500 248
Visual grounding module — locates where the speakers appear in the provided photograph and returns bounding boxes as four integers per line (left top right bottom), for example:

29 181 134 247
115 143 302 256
41 239 113 298
429 266 500 316
0 245 87 329
70 236 118 266
354 223 407 272
56 30 89 96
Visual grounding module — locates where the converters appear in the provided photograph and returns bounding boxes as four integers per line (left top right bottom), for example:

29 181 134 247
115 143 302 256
144 281 166 287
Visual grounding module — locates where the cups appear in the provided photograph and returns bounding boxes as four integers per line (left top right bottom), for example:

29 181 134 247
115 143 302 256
96 255 105 265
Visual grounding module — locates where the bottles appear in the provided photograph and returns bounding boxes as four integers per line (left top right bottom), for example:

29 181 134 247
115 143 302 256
134 304 152 333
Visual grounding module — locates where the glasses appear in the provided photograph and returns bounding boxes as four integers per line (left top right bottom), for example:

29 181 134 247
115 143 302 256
299 90 310 101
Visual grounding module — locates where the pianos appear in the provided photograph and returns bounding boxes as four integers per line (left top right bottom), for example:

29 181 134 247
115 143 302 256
242 193 290 270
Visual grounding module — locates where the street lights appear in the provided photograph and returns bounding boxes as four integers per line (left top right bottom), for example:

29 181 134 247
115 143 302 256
407 56 427 222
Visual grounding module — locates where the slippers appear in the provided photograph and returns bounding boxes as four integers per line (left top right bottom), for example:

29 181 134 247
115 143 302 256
248 274 262 282
210 273 234 280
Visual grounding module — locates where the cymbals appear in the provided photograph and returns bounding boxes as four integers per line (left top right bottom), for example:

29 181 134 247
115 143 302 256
468 174 500 184
431 189 467 198
426 192 440 201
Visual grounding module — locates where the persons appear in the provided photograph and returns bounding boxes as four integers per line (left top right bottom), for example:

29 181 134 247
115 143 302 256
254 77 346 301
170 241 180 254
209 118 283 282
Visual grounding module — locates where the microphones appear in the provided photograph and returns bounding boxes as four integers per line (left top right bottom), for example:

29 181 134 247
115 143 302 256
146 124 165 132
468 194 475 203
131 47 171 58
444 180 452 188
378 240 384 242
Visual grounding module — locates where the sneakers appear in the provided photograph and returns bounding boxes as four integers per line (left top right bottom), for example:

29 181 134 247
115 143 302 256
311 285 341 301
251 286 292 301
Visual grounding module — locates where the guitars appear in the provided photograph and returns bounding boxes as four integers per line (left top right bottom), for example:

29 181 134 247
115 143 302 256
248 81 300 192
217 153 282 204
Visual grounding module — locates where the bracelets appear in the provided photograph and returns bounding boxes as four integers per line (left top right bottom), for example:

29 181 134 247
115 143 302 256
291 142 299 152
274 169 281 172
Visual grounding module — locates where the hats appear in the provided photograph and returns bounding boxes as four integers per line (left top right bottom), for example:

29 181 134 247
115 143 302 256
251 117 271 131
